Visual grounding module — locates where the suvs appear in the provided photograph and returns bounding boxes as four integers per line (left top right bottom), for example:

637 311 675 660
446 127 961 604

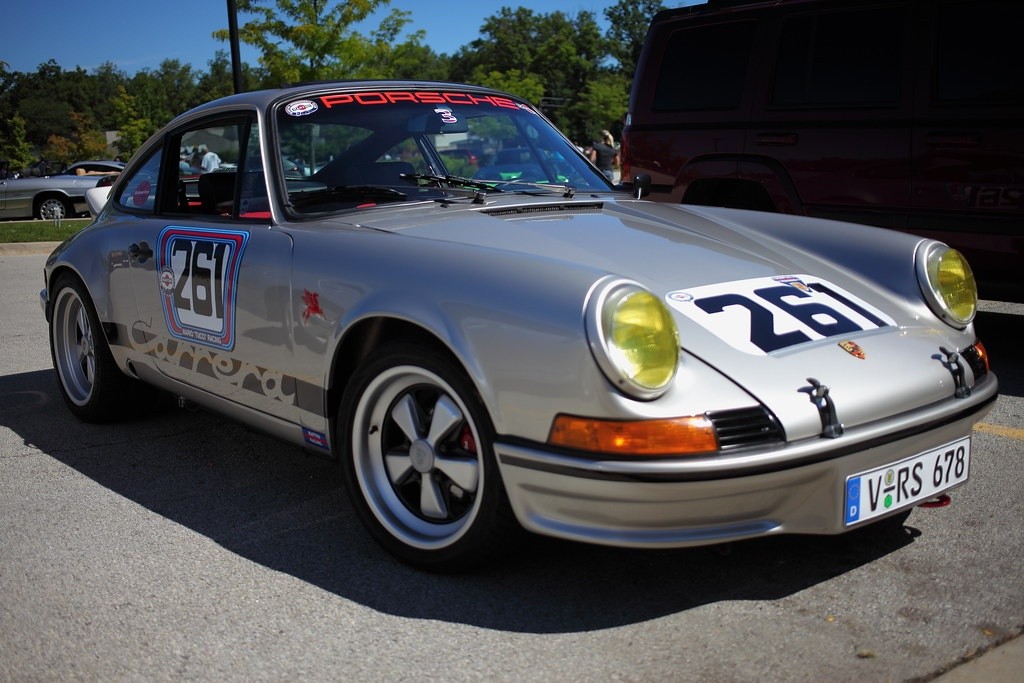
612 0 1024 313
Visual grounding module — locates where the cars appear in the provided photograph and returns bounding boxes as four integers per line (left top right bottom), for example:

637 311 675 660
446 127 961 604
0 157 327 223
37 77 1002 576
472 145 562 184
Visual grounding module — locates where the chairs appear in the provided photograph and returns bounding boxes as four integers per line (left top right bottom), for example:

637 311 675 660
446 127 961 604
197 171 267 214
76 168 86 175
336 162 420 201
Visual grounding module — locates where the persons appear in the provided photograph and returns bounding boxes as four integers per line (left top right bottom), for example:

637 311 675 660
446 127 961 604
574 142 621 170
588 130 620 181
198 145 221 173
179 154 190 169
